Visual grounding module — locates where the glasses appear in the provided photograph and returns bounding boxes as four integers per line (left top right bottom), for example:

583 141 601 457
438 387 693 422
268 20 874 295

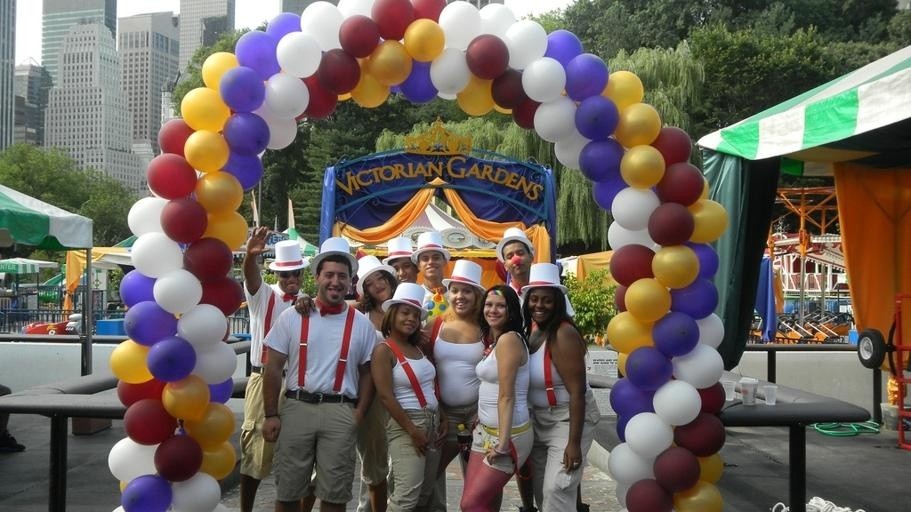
276 271 302 277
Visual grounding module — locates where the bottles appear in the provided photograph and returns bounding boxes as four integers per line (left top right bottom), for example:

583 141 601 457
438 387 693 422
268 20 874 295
456 422 472 457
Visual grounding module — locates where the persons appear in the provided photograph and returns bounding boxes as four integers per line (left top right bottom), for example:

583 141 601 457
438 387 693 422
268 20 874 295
240 227 600 512
4 273 18 308
0 386 26 452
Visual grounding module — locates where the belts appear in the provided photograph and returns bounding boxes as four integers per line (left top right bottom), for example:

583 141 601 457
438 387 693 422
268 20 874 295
284 390 351 405
249 365 287 376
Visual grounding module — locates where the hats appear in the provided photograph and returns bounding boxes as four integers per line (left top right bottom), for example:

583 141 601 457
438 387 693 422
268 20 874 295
496 227 534 263
521 262 568 296
312 232 487 320
268 240 310 272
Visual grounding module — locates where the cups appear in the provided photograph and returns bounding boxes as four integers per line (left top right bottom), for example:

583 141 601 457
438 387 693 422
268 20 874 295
720 380 735 402
740 380 759 406
761 384 777 407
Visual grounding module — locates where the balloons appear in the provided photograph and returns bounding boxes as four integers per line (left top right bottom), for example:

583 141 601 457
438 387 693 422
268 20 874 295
403 1 727 182
591 173 728 512
108 0 439 511
